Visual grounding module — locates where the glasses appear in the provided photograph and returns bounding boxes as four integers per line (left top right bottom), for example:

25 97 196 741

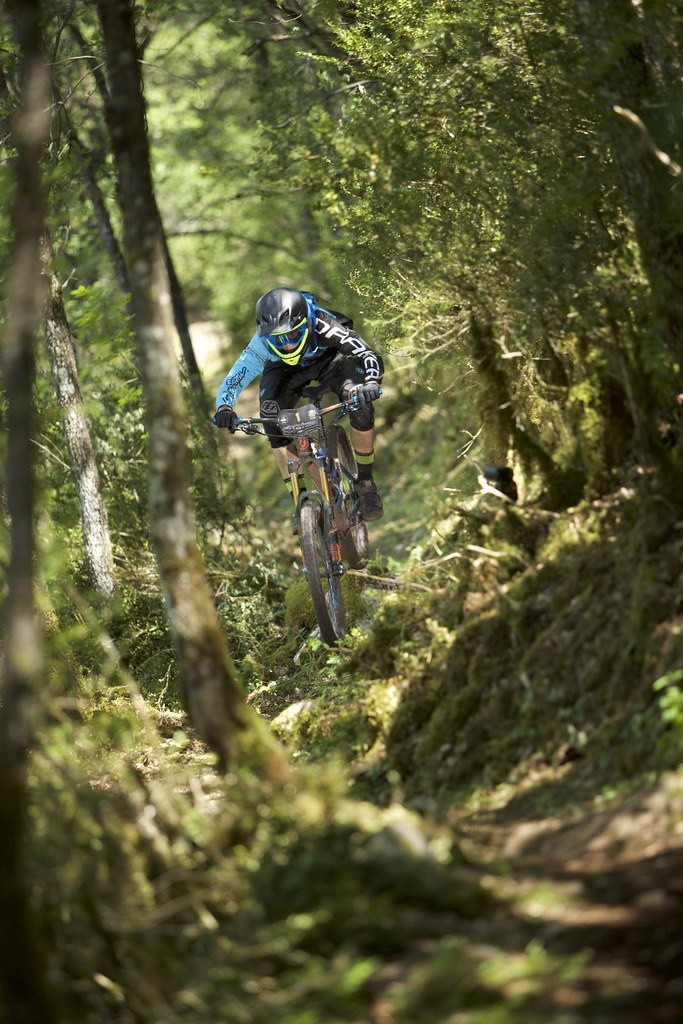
265 320 311 350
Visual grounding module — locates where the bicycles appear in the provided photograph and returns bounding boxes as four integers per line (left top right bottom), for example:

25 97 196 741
213 389 385 648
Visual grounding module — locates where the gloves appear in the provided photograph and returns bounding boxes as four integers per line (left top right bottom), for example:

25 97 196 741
357 381 380 410
215 409 238 434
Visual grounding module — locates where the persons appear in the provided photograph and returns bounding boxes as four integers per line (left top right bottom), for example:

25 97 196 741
216 287 383 535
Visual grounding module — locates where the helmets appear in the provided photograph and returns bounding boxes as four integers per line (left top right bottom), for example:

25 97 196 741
256 287 316 366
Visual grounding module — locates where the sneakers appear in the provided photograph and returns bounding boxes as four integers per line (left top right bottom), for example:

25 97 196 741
290 507 300 535
355 476 384 522
